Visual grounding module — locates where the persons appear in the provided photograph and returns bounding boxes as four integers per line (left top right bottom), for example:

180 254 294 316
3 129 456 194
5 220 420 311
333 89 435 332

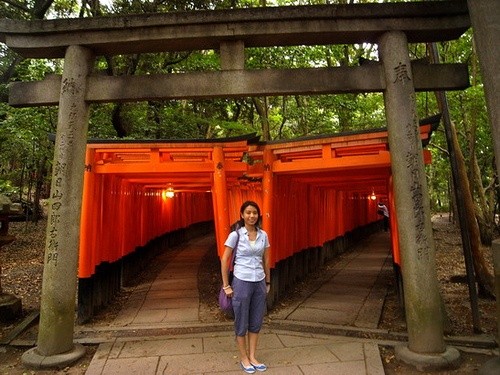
221 201 270 374
378 202 389 231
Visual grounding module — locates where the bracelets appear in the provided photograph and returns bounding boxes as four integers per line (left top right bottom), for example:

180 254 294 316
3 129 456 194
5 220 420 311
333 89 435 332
266 282 271 285
223 285 230 289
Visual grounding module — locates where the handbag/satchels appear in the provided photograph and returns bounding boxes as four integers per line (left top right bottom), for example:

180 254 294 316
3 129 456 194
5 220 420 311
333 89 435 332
217 285 234 315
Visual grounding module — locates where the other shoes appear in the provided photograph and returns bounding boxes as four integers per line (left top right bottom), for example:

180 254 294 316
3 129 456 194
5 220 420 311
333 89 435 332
240 359 267 374
